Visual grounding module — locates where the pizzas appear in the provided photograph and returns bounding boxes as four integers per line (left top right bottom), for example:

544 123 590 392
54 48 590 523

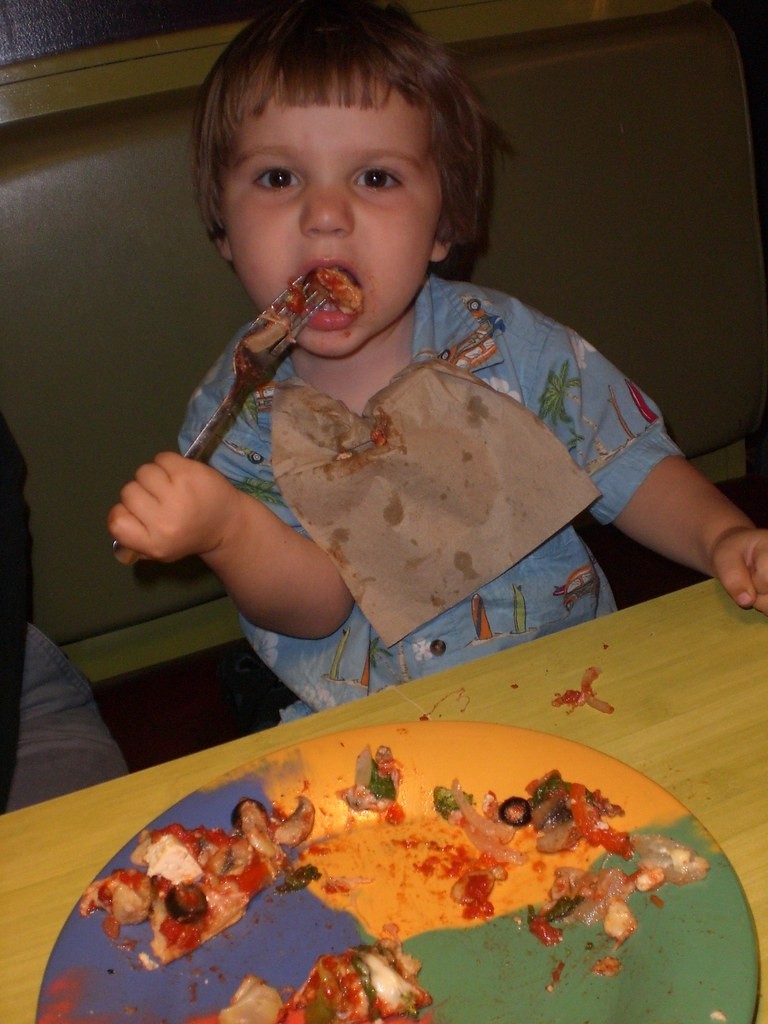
139 797 315 962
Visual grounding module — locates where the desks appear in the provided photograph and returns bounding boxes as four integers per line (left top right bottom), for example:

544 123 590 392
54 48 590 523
0 579 768 1024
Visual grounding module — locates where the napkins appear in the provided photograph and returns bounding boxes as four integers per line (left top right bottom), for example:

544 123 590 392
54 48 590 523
270 359 604 649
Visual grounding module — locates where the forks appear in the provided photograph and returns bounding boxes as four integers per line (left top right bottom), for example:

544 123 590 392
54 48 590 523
108 277 328 569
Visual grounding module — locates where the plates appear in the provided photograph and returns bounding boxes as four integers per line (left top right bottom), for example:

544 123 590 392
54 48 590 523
36 721 760 1024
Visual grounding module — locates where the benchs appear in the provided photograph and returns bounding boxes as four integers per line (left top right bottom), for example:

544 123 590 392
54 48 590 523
0 0 768 802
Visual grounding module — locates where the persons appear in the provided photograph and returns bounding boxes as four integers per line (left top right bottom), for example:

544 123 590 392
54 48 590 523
0 411 129 813
108 0 768 707
